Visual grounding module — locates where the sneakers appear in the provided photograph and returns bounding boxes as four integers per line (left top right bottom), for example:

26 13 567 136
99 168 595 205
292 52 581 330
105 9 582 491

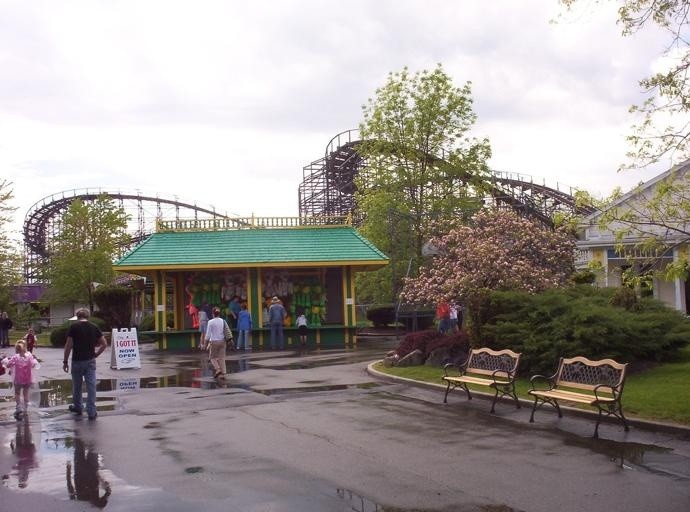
69 405 82 416
214 370 227 386
13 409 31 418
89 412 98 421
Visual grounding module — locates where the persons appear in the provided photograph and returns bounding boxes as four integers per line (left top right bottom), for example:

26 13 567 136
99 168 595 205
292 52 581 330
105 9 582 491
1 339 41 417
437 302 449 336
23 328 37 353
62 307 108 419
197 295 309 379
448 300 460 334
0 311 12 348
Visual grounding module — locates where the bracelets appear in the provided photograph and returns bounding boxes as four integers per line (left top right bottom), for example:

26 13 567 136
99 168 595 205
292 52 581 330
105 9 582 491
63 360 68 364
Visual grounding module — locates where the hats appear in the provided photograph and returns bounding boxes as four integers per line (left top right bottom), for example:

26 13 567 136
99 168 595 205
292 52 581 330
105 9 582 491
271 297 280 304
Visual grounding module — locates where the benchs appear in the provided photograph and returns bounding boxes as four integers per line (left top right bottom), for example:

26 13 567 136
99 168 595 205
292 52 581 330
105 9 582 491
441 347 523 414
527 354 631 438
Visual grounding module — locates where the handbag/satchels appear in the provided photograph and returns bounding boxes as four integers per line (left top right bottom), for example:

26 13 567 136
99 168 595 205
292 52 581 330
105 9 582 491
223 320 234 342
